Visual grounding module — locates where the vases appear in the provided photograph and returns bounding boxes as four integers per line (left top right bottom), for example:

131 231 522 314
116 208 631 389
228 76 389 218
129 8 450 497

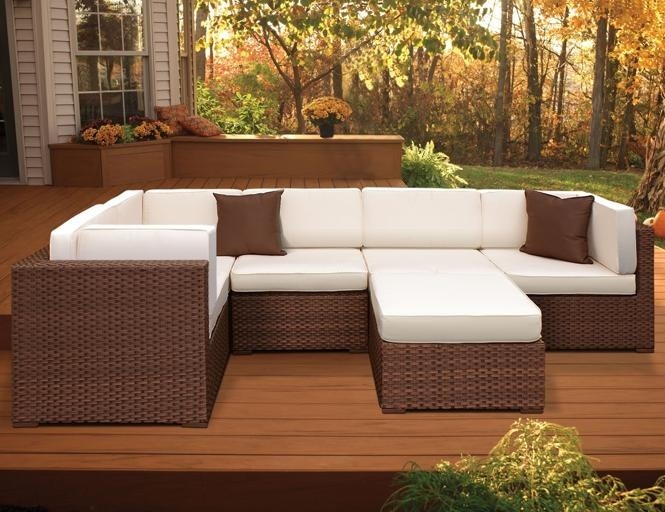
320 123 334 138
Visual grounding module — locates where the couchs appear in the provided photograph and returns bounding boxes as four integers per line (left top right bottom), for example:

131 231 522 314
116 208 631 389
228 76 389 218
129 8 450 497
12 187 654 427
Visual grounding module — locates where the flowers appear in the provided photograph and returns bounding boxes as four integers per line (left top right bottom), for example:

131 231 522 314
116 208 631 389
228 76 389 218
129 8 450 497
78 114 172 147
300 95 354 128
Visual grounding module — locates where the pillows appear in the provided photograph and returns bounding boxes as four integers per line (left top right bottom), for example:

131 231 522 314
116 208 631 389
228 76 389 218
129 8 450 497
212 189 288 256
154 103 224 138
519 188 595 265
